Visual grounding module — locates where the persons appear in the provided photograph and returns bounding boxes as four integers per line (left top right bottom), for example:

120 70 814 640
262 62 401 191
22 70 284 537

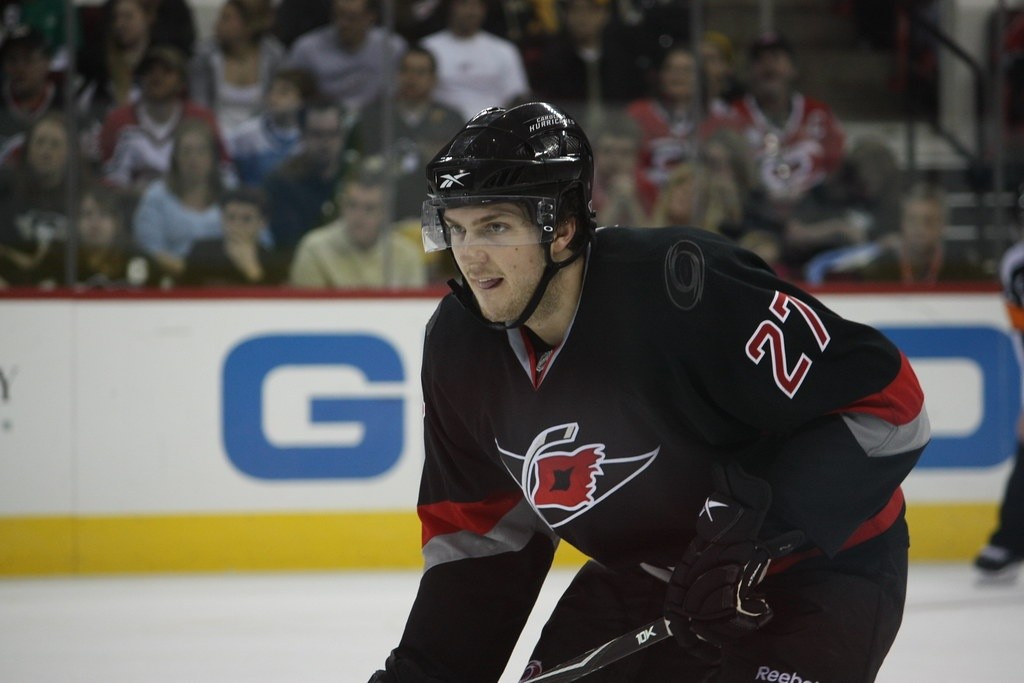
973 187 1024 569
367 99 934 683
0 0 944 297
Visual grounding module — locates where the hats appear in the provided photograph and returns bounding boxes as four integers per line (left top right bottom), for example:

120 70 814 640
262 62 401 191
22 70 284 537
752 31 785 48
0 25 38 48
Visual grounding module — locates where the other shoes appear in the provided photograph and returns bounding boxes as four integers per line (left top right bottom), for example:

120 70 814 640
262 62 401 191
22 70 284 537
975 544 1017 570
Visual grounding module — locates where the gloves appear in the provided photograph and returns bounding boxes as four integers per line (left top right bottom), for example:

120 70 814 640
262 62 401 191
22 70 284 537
664 492 810 667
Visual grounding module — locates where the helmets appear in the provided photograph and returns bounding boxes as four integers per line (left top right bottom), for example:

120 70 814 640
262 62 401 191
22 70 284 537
424 102 596 227
